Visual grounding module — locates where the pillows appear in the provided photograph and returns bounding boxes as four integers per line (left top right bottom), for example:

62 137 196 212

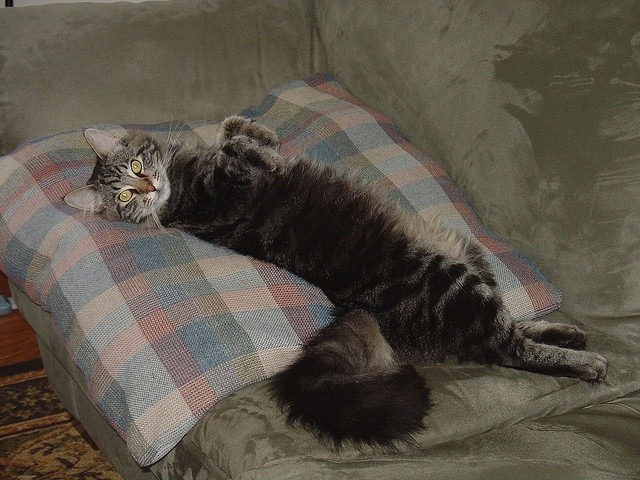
0 71 565 469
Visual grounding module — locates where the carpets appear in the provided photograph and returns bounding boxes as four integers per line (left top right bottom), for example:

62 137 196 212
0 357 127 480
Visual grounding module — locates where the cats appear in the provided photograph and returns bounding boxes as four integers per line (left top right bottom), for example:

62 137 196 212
63 115 609 455
